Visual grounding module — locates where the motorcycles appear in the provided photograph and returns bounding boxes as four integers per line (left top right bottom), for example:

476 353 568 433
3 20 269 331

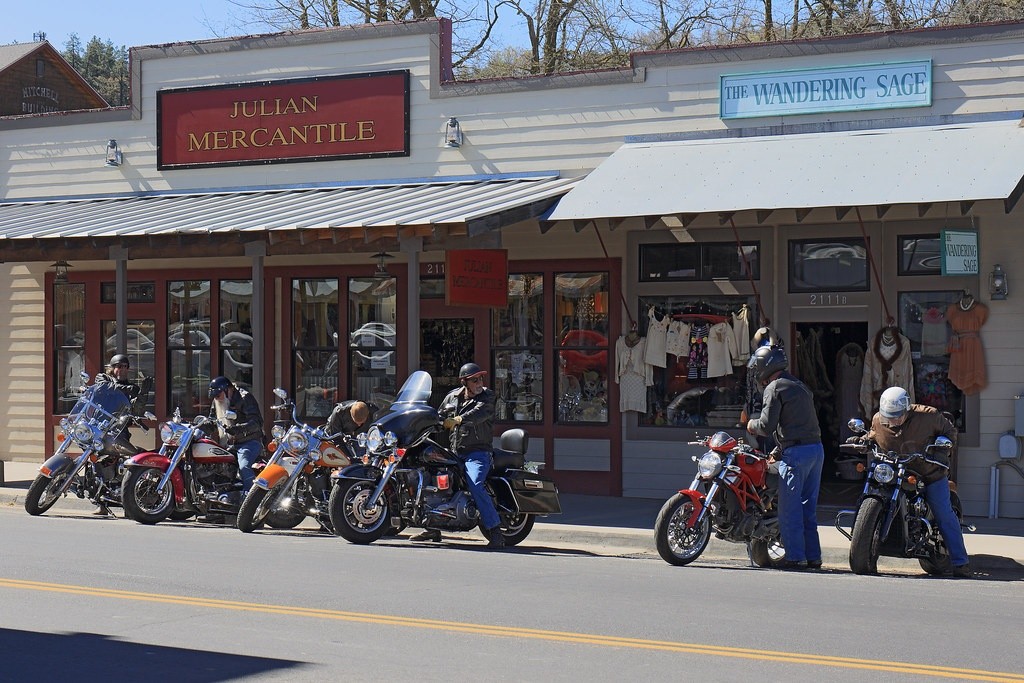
25 373 414 539
834 417 977 576
327 371 564 545
654 431 796 570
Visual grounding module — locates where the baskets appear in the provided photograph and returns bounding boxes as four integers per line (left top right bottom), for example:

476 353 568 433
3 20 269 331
833 455 865 481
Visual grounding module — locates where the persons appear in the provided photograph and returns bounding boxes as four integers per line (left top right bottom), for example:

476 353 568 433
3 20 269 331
196 377 267 524
742 385 786 462
846 387 975 578
94 353 155 515
744 343 824 571
407 363 507 549
666 386 734 428
64 339 85 393
318 399 379 533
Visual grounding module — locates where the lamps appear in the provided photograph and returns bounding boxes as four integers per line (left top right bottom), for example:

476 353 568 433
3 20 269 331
444 117 463 146
50 259 74 282
105 140 122 165
369 250 395 278
988 264 1009 300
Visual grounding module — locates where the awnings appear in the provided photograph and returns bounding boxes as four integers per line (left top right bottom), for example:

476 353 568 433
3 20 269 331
0 163 590 425
537 118 1024 331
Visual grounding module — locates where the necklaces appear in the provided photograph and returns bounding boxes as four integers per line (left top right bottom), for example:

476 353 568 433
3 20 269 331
874 326 903 379
960 297 974 310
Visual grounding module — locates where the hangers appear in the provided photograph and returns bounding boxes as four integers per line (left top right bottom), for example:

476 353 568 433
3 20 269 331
959 289 973 301
884 318 894 331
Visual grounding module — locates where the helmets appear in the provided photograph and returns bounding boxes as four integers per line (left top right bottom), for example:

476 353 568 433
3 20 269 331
206 376 233 398
108 354 130 374
745 344 788 386
458 362 488 382
879 386 912 426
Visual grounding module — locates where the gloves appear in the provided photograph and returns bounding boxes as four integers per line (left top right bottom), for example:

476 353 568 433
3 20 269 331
127 384 140 398
140 377 154 397
933 450 949 474
845 435 862 445
225 424 240 435
442 415 462 432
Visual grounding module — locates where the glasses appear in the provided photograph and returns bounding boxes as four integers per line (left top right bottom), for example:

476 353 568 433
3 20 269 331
113 364 128 369
464 376 483 383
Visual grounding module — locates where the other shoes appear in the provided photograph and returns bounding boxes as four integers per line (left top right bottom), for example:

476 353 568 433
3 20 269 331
806 559 822 572
485 526 506 549
769 558 808 571
411 530 442 542
951 564 974 578
196 515 226 524
